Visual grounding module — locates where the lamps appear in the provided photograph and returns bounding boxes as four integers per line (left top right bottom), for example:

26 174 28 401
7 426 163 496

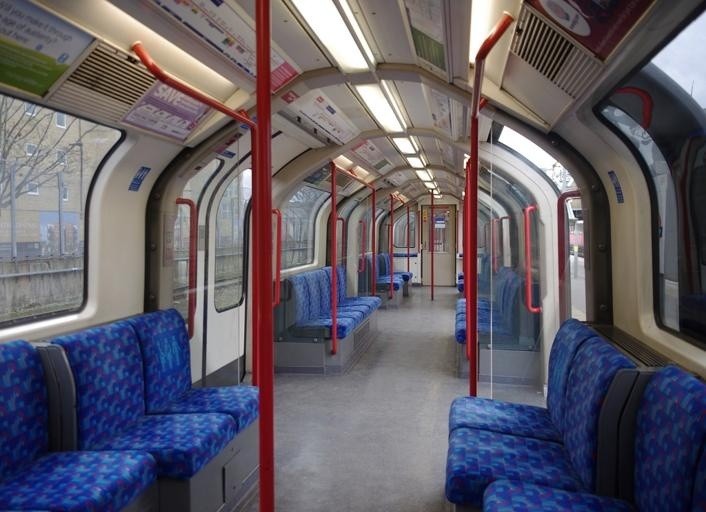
283 0 443 199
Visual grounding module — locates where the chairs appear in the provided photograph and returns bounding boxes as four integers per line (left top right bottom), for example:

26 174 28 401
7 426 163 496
455 266 540 380
0 338 160 512
458 255 491 292
358 252 413 303
125 308 259 511
50 320 237 512
275 264 382 376
